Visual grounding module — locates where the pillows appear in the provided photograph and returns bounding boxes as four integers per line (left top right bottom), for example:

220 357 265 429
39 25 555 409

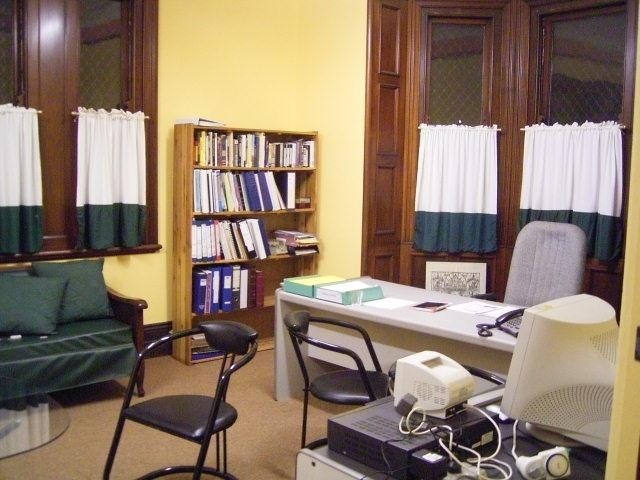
0 273 70 337
31 258 116 325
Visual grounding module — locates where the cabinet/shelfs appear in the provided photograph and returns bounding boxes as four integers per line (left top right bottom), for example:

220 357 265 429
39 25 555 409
173 123 320 367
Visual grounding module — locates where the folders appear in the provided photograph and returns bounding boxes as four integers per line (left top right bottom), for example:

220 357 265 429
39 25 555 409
245 172 296 212
192 266 248 316
174 117 228 126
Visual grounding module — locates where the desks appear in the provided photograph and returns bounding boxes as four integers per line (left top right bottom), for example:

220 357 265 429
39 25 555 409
296 369 540 479
274 272 530 403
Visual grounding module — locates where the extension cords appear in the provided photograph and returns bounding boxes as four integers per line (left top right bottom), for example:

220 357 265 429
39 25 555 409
442 461 488 480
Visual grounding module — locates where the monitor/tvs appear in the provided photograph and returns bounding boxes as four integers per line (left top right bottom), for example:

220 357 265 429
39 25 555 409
500 292 621 453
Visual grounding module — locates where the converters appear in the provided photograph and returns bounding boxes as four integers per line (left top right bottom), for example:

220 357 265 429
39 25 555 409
410 449 448 480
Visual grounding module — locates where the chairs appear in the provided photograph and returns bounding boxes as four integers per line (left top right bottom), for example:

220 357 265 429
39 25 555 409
471 219 588 310
283 310 392 448
101 320 260 480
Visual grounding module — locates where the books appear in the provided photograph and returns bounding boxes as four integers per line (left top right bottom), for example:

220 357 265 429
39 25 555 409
194 130 318 316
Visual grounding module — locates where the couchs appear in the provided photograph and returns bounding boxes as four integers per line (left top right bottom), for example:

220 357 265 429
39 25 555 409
0 263 148 402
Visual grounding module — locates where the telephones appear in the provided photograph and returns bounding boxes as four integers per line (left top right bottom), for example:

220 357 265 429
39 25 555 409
494 308 527 338
